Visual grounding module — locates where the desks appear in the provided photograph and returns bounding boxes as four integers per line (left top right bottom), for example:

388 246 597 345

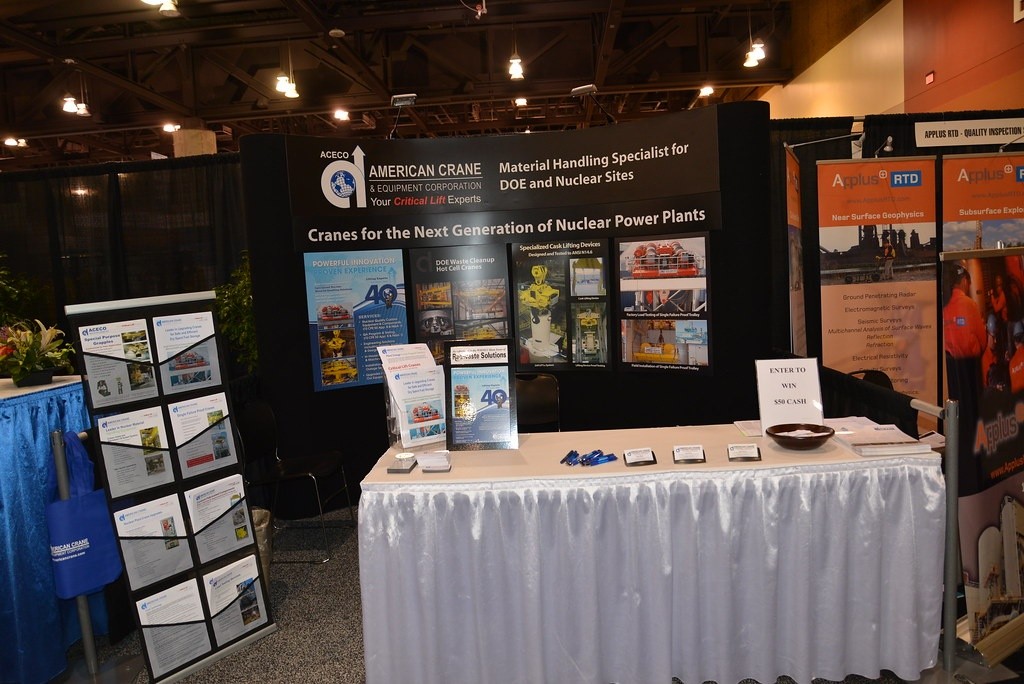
353 425 947 684
0 375 135 684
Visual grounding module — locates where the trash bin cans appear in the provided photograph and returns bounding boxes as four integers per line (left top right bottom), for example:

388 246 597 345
251 509 272 598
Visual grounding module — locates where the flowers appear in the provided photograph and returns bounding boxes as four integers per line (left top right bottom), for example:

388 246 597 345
0 319 77 383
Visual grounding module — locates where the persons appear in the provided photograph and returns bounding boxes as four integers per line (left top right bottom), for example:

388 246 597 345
943 267 1024 462
882 241 896 279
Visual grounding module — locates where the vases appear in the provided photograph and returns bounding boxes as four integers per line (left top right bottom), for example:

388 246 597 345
14 367 55 388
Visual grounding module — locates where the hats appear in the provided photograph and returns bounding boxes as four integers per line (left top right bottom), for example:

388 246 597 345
951 264 971 283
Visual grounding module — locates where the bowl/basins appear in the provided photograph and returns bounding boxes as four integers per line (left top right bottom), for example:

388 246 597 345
766 424 835 451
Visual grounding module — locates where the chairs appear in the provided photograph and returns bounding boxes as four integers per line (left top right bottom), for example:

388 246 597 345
226 373 357 565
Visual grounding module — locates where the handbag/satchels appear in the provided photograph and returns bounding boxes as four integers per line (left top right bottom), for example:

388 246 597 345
45 432 122 599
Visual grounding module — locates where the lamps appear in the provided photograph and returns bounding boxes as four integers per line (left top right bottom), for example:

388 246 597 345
62 69 92 117
743 10 766 67
275 46 299 98
159 0 181 17
385 94 417 139
508 15 524 81
571 84 617 125
5 138 29 148
515 99 527 107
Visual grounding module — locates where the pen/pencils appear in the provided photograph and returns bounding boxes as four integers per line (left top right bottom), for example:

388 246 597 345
559 448 618 467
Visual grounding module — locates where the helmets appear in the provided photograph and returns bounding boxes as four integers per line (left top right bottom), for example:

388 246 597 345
1013 322 1024 335
987 315 997 333
